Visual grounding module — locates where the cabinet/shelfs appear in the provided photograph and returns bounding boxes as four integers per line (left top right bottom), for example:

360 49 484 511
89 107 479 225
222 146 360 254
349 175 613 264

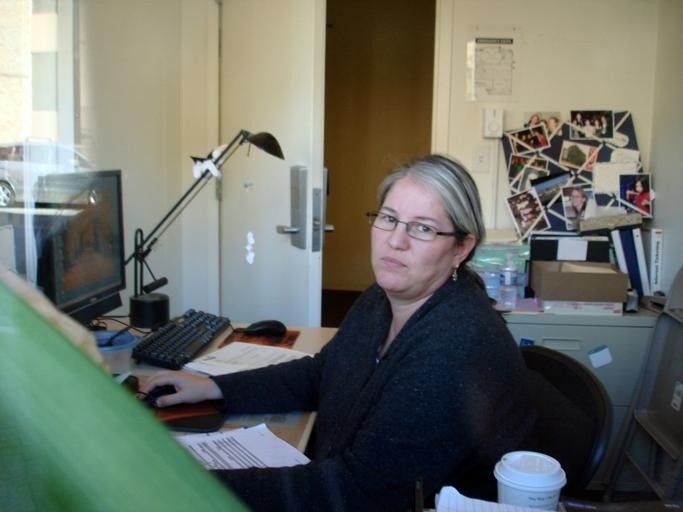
498 307 659 492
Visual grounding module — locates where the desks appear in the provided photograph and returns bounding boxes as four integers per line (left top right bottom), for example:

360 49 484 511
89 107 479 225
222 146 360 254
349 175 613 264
88 315 339 453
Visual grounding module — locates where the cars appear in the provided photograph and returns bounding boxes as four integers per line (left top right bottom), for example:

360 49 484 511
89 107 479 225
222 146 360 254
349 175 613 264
0 141 108 210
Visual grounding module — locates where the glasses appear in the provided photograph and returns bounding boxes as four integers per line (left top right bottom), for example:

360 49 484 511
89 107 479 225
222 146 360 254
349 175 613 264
366 210 459 241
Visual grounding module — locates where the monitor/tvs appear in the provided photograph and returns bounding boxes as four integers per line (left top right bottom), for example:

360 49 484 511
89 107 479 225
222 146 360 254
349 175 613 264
36 170 143 353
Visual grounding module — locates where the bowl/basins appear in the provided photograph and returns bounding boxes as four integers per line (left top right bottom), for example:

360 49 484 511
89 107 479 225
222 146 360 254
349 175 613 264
90 328 139 375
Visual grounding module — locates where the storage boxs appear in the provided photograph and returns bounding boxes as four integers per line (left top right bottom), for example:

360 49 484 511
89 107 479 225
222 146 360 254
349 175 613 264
532 261 628 301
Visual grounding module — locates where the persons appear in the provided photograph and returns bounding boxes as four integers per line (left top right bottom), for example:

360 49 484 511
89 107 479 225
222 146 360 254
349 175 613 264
133 155 547 512
503 106 652 239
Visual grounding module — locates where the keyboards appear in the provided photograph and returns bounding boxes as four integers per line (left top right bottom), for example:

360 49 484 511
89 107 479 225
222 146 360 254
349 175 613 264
133 308 231 369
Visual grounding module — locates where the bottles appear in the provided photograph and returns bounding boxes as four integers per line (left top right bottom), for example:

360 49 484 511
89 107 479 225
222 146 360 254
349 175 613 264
498 251 519 310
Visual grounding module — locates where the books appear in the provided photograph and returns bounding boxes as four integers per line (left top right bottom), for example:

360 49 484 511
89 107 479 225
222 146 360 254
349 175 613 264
182 341 315 377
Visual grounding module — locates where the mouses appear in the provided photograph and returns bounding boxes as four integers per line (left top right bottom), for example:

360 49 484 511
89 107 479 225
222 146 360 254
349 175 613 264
246 320 286 334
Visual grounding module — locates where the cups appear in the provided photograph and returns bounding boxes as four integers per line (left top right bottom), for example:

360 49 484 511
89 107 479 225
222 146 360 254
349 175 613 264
493 451 568 512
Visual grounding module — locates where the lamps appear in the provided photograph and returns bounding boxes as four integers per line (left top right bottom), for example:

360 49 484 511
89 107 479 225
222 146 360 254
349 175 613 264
123 130 284 328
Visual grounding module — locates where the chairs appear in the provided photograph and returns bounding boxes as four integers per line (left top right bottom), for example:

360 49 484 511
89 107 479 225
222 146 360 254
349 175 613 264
477 345 613 504
